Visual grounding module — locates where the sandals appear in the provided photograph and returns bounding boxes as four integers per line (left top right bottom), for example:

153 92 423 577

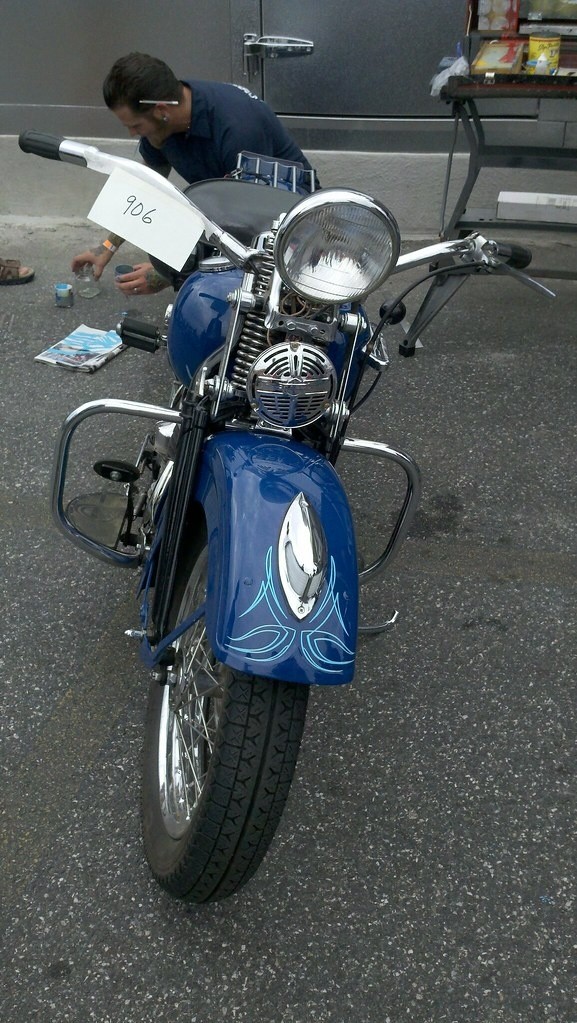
0 256 35 285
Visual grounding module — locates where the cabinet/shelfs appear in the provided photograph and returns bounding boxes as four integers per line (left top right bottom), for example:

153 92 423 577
399 0 577 357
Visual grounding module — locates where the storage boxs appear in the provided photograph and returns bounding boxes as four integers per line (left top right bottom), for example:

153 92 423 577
496 191 577 223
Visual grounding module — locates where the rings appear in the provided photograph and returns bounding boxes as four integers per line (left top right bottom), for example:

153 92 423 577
134 288 138 295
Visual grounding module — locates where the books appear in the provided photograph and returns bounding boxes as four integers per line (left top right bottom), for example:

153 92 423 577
35 324 127 373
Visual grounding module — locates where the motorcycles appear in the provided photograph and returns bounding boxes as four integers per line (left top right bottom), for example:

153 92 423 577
15 129 559 904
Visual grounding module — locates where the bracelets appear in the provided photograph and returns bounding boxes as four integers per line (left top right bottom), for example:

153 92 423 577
103 240 118 253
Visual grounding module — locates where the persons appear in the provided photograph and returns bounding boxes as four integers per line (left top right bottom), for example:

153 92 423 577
0 257 35 286
70 52 321 295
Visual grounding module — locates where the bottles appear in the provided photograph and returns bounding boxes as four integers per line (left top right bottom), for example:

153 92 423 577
78 266 100 298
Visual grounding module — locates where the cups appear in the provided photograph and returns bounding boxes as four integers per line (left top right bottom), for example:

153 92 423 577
525 30 561 75
55 282 74 309
114 265 134 289
